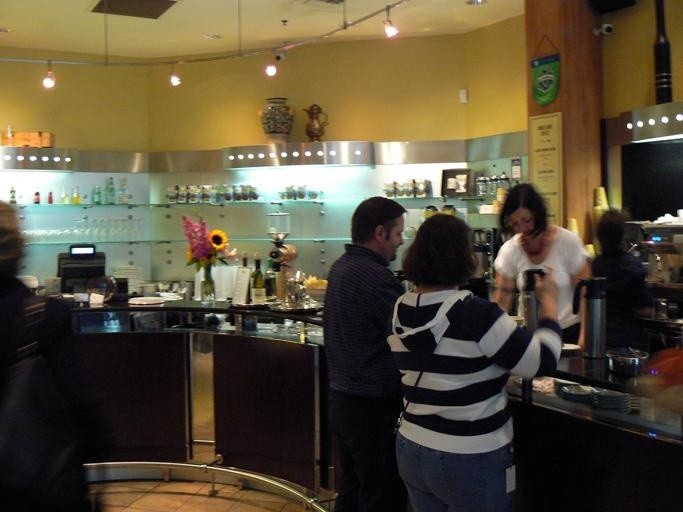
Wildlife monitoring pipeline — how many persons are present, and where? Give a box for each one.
[492,183,592,350]
[322,196,410,511]
[592,209,660,347]
[1,203,77,511]
[387,214,563,512]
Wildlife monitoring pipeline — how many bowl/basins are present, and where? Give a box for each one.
[601,348,650,376]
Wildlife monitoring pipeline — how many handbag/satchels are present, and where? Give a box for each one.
[0,341,108,511]
[368,432,398,473]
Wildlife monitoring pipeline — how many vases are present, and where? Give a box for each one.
[258,98,294,143]
[201,282,216,304]
[183,214,237,304]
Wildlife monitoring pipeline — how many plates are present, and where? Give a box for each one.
[553,379,640,414]
[117,267,157,297]
[128,297,165,305]
[562,343,580,352]
[162,291,183,301]
[269,302,325,314]
[231,302,269,309]
[305,288,328,301]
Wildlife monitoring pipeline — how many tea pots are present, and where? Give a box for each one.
[572,276,610,359]
[519,269,546,330]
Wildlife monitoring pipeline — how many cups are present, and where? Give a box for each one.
[653,298,672,320]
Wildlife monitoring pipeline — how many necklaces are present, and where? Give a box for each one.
[414,287,441,293]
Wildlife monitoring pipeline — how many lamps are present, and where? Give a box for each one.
[0,0,402,88]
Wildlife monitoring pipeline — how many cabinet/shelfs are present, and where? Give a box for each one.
[375,129,528,292]
[630,224,683,353]
[1,140,375,290]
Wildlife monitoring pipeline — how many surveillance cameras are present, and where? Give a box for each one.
[595,22,615,39]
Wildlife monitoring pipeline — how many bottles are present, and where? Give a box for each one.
[243,251,248,266]
[475,172,512,196]
[286,269,306,308]
[249,252,273,302]
[9,177,129,204]
